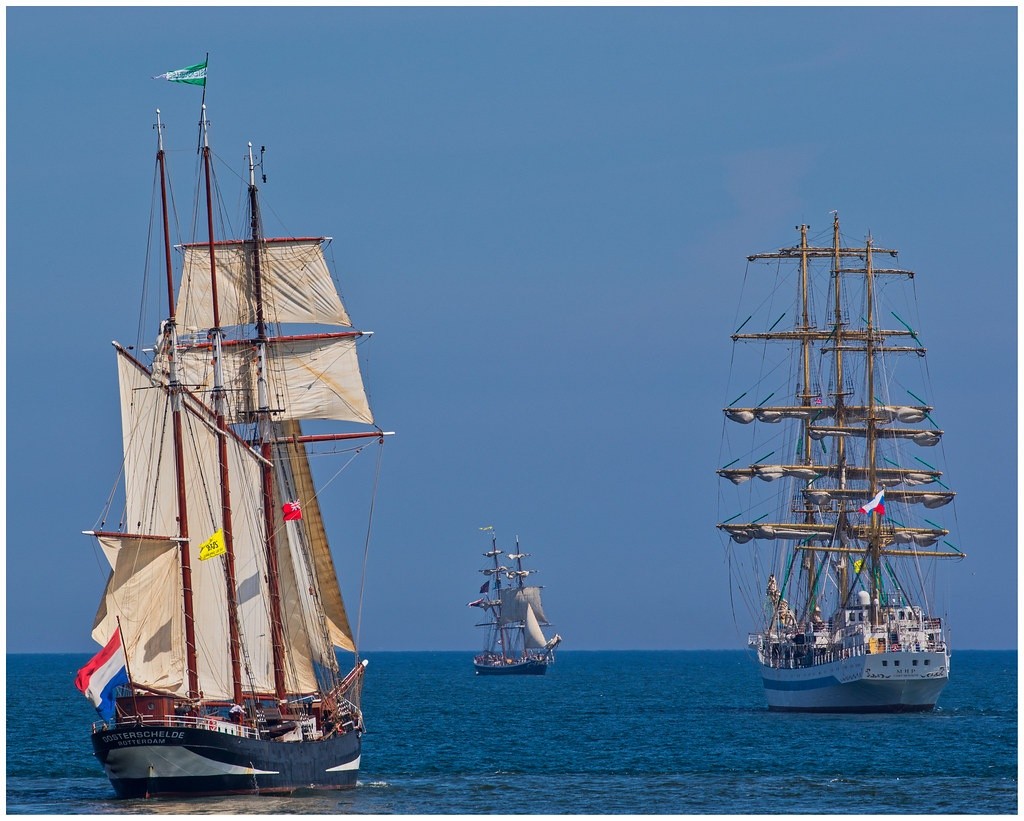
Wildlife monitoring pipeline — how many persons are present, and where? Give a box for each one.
[321,710,338,739]
[173,702,195,727]
[227,701,249,724]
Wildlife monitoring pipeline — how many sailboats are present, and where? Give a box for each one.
[718,206,969,714]
[465,526,563,676]
[79,102,396,802]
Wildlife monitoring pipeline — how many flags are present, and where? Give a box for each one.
[76,625,128,729]
[480,583,489,594]
[853,558,867,573]
[468,598,484,608]
[197,529,226,563]
[859,488,885,516]
[280,500,302,519]
